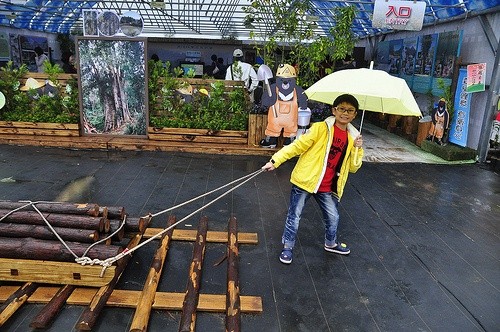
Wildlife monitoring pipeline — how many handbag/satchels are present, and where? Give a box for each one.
[213,66,219,74]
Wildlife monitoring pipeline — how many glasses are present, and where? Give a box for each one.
[333,106,356,114]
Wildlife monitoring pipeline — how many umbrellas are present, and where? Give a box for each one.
[302,60,424,167]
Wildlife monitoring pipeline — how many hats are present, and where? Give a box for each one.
[232,48,243,57]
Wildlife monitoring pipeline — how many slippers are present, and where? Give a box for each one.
[324,242,351,255]
[279,245,293,264]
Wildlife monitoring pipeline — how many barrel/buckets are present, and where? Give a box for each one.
[298,107,311,126]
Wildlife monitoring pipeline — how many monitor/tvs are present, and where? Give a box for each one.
[180,62,205,78]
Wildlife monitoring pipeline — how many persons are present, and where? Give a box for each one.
[224,48,259,105]
[34,46,52,74]
[61,51,77,74]
[205,54,226,79]
[261,94,364,264]
